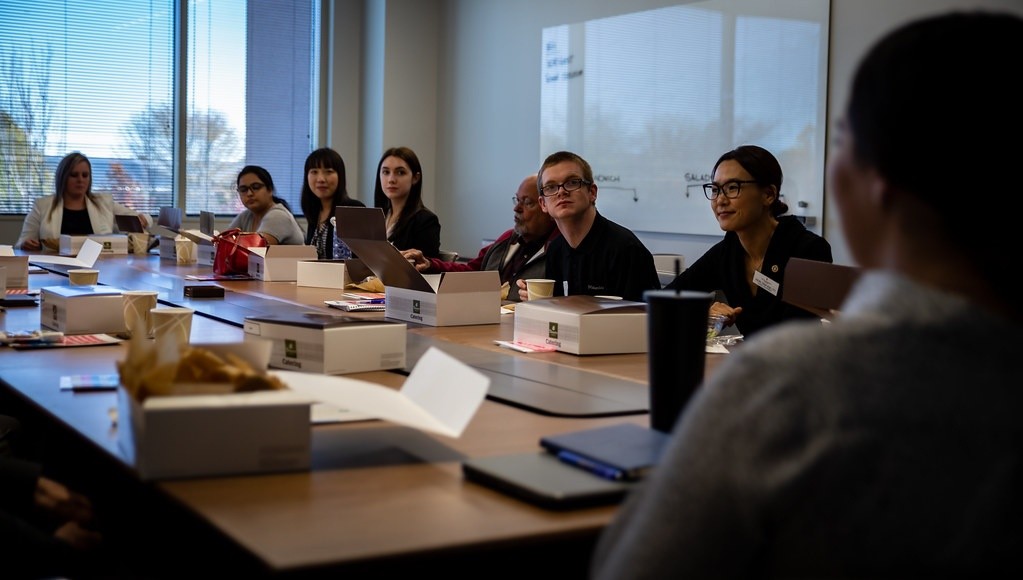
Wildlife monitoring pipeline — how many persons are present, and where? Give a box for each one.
[15,153,153,248]
[664,143,833,344]
[394,176,562,302]
[228,167,306,246]
[302,148,367,258]
[369,147,442,264]
[536,150,662,301]
[587,10,1023,580]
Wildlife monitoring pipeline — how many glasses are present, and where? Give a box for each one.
[541,178,590,196]
[237,182,266,194]
[702,179,753,200]
[511,197,537,209]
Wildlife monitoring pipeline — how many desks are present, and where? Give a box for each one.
[0,247,744,570]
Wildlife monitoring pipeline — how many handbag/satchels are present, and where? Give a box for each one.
[211,227,267,275]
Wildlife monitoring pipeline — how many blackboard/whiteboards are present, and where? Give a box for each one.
[538,0,831,239]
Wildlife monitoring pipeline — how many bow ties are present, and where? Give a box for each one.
[510,234,524,245]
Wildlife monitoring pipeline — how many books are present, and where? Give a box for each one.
[324,290,385,311]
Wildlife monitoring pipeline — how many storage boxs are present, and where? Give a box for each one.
[243,311,408,376]
[146,207,219,266]
[297,258,374,289]
[782,258,855,321]
[59,232,129,256]
[211,234,319,283]
[0,245,29,290]
[514,294,648,355]
[40,286,125,334]
[117,336,493,480]
[335,206,503,327]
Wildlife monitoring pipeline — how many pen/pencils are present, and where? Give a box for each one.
[556,450,623,482]
[356,298,385,305]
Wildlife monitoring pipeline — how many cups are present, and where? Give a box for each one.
[175,238,195,267]
[68,269,101,286]
[121,290,159,339]
[525,278,556,301]
[132,232,149,256]
[150,307,196,345]
[643,290,714,434]
